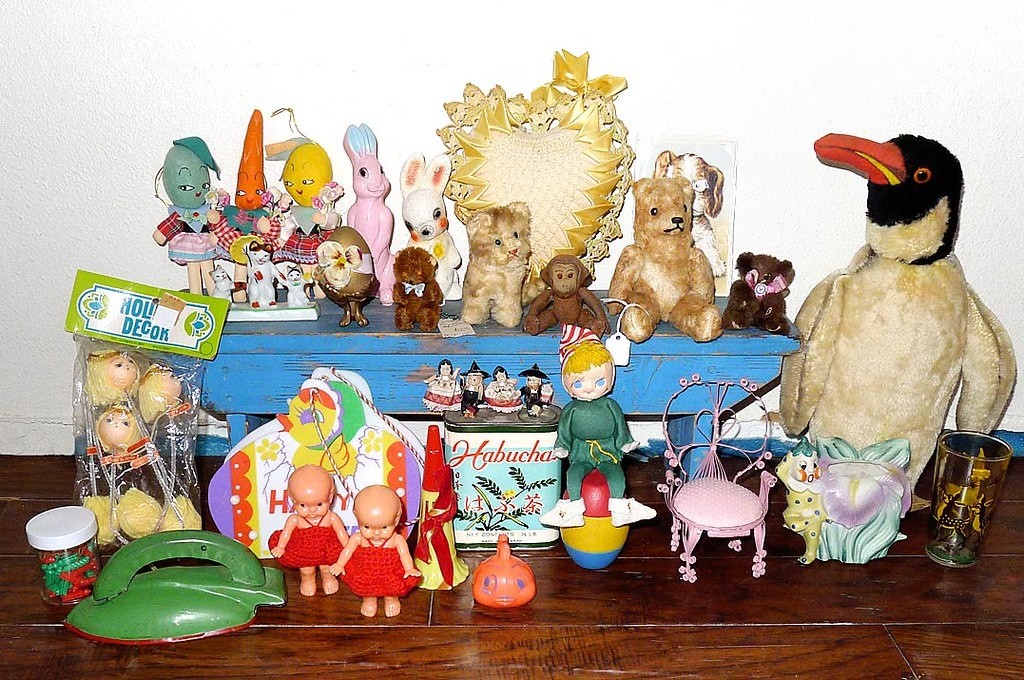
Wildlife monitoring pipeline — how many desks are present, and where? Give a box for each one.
[141,286,801,497]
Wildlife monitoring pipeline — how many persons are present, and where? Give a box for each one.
[264,465,348,598]
[539,322,659,530]
[328,484,423,618]
[420,358,552,420]
[67,345,205,542]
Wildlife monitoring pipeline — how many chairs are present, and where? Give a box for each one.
[656,375,780,585]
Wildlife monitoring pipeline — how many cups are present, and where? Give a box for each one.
[925,431,1012,568]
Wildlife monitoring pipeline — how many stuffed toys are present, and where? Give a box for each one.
[773,132,1018,493]
[156,109,797,338]
